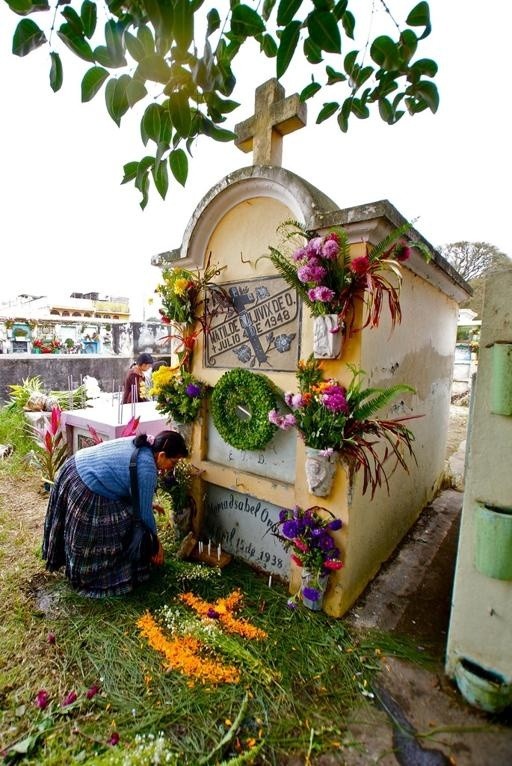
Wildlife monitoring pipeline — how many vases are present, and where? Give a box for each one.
[312,315,344,360]
[305,447,337,497]
[301,567,331,610]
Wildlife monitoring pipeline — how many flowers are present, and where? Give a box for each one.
[278,506,344,611]
[154,253,238,367]
[266,352,425,500]
[149,367,210,421]
[269,215,432,337]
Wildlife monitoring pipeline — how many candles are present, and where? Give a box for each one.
[196,537,222,562]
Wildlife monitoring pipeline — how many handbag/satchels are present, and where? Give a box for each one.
[123,518,159,569]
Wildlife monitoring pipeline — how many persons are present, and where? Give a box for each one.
[42,430,188,600]
[145,361,167,402]
[121,353,150,404]
